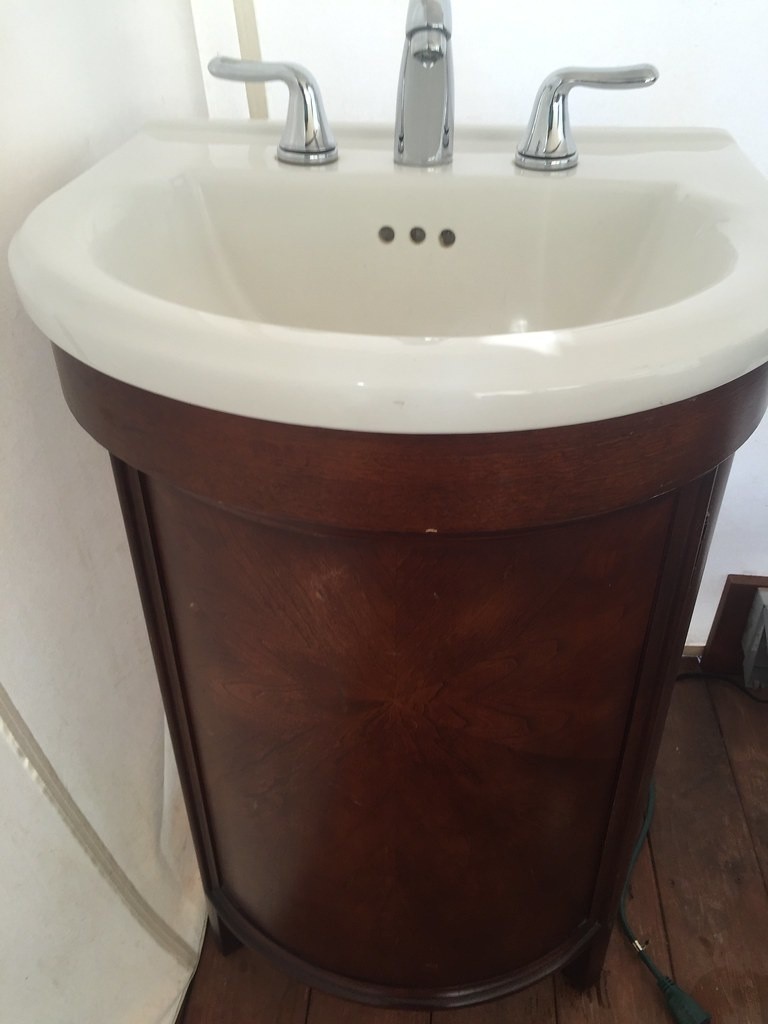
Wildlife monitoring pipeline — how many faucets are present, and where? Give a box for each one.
[390,0,455,170]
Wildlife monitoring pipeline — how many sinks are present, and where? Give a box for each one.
[4,115,764,437]
